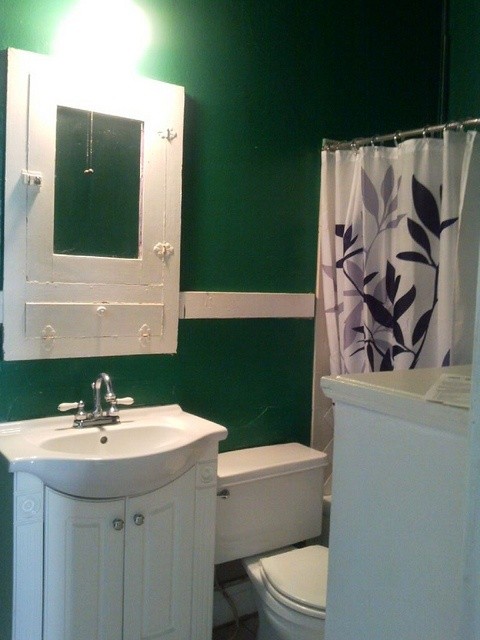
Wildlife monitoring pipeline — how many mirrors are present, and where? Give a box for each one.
[51,104,148,262]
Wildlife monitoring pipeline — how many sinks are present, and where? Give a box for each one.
[0,403,228,473]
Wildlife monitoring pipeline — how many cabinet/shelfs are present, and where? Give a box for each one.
[318,360,470,640]
[9,463,216,639]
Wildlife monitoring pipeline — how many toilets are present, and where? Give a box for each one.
[216,439,329,640]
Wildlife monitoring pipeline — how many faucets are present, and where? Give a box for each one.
[86,368,119,426]
